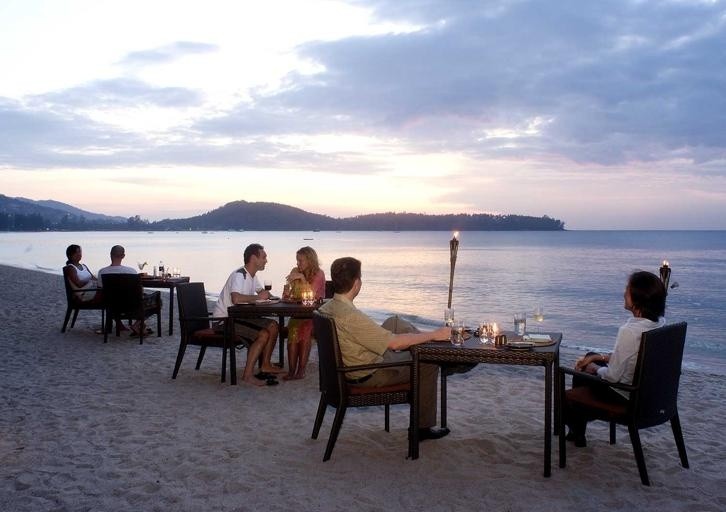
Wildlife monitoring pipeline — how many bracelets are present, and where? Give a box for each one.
[598,352,603,360]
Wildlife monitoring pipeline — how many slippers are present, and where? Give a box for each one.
[267,378,279,386]
[130,328,154,337]
[254,372,277,380]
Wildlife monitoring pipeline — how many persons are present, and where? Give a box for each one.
[64,244,130,331]
[282,246,325,380]
[209,245,287,386]
[562,271,669,447]
[319,258,479,442]
[96,246,153,337]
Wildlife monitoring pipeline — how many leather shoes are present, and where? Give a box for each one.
[408,427,449,441]
[447,363,478,375]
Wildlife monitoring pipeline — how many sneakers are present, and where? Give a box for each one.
[567,431,586,448]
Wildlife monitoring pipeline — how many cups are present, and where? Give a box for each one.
[444,307,454,328]
[264,280,272,293]
[305,290,313,303]
[533,303,544,336]
[479,320,488,345]
[451,320,465,347]
[488,321,498,343]
[301,289,308,304]
[513,311,527,337]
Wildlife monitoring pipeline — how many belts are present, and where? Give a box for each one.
[346,370,378,384]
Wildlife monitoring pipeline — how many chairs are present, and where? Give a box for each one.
[172,281,246,384]
[285,280,335,339]
[59,265,105,335]
[556,320,691,488]
[98,273,162,346]
[310,309,418,463]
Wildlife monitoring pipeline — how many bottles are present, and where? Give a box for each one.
[502,335,507,344]
[152,266,157,278]
[284,284,289,299]
[159,260,164,277]
[495,336,500,345]
